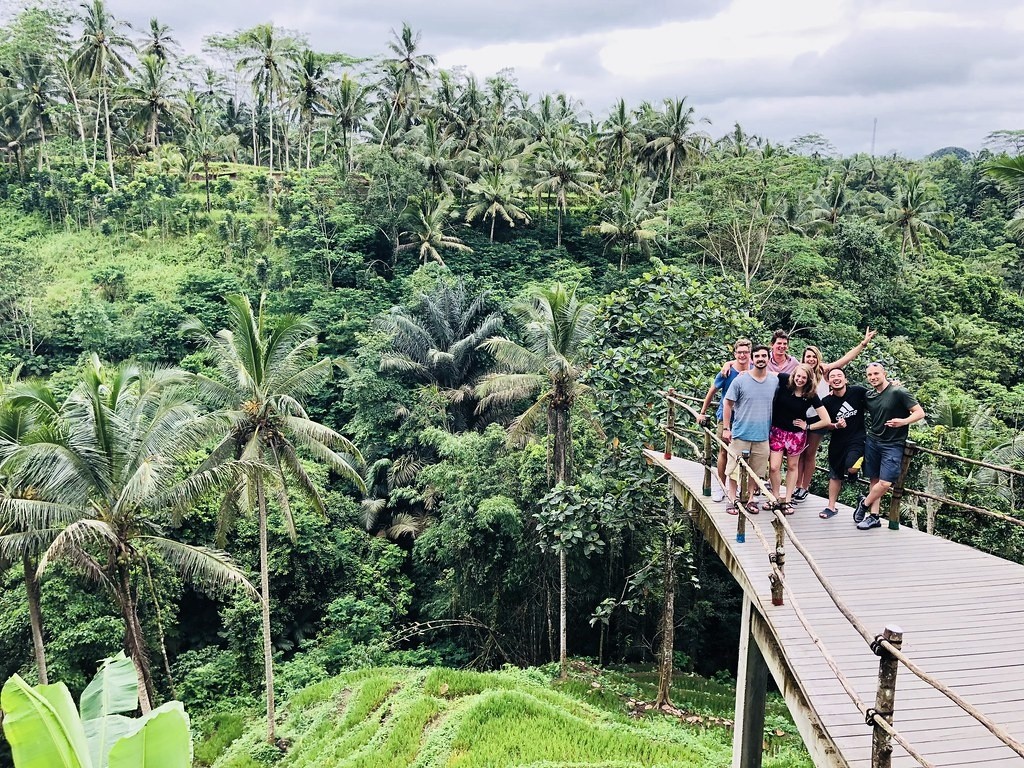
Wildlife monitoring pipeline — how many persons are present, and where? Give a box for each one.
[721,330,800,379]
[792,327,879,503]
[818,368,901,519]
[697,339,761,502]
[739,364,831,515]
[853,363,925,530]
[723,347,779,515]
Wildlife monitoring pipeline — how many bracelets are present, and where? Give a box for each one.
[861,343,867,347]
[806,424,810,430]
[723,428,730,430]
[700,413,705,415]
[834,424,838,429]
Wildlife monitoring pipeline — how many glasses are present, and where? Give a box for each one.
[735,351,749,355]
[865,363,878,371]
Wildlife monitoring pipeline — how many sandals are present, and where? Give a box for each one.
[753,485,772,495]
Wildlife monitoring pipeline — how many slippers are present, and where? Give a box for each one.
[726,500,759,515]
[849,472,859,484]
[763,500,794,515]
[819,507,839,520]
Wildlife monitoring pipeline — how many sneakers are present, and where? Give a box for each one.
[712,488,741,502]
[854,496,882,530]
[791,487,809,503]
